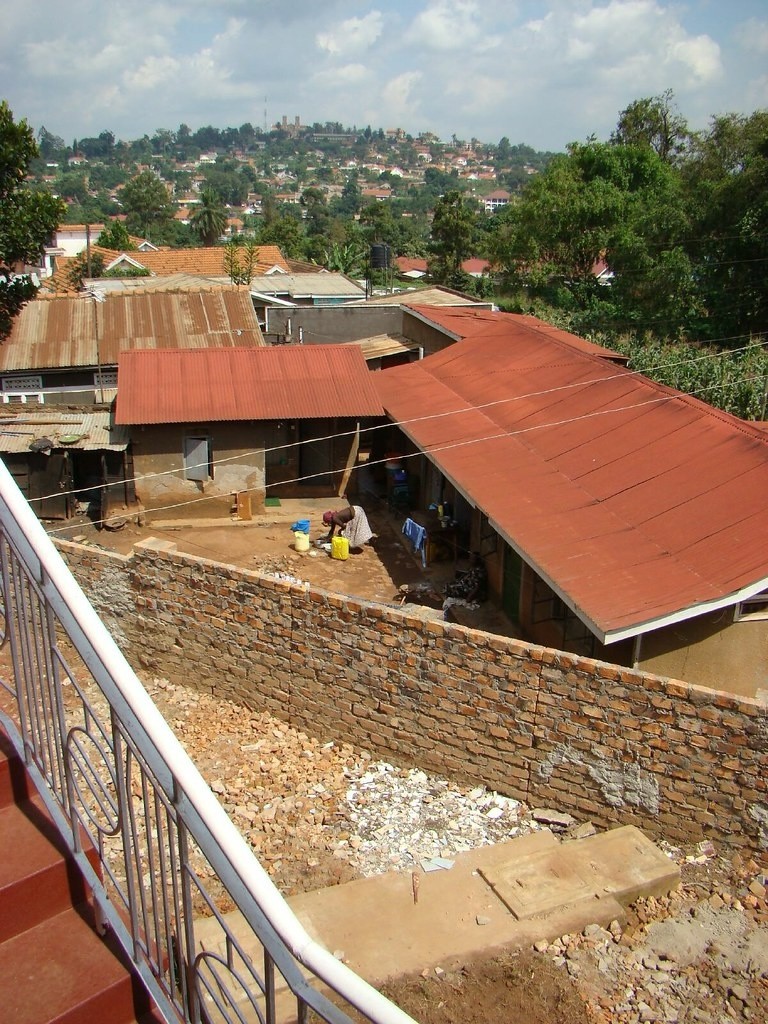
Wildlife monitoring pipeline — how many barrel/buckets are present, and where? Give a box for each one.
[296,519,310,533]
[331,536,349,560]
[294,531,309,551]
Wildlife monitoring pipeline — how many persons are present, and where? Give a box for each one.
[446,551,486,603]
[323,506,374,549]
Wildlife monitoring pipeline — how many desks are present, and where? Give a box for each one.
[410,508,460,567]
[384,466,420,520]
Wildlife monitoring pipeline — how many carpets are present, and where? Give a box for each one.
[265,497,283,507]
